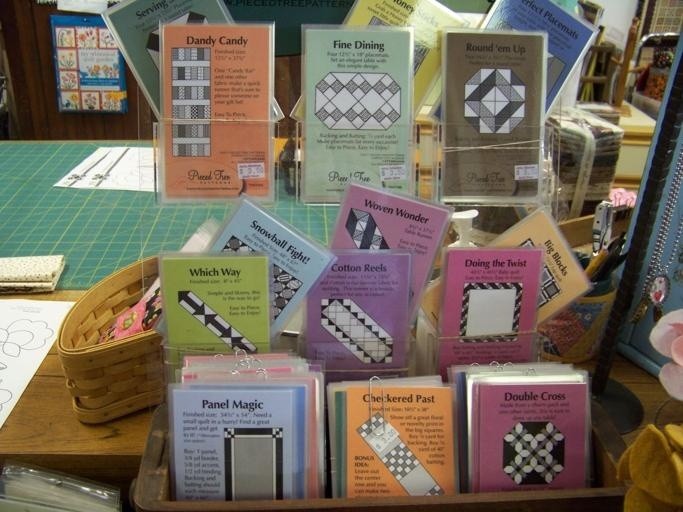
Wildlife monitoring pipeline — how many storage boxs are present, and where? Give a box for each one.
[303,341,417,383]
[133,397,627,511]
[153,125,275,205]
[614,101,655,188]
[417,331,540,375]
[161,343,278,385]
[435,126,552,205]
[294,123,419,210]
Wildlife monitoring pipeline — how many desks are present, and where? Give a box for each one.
[0,139,683,511]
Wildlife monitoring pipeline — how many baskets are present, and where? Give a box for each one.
[57,256,169,425]
